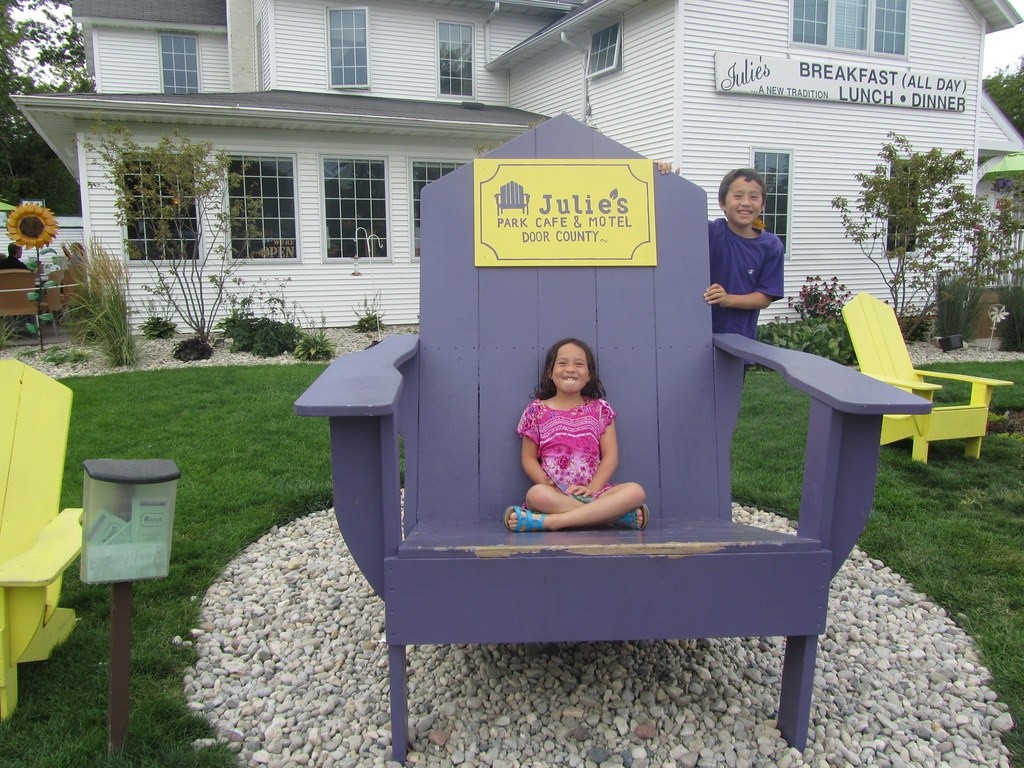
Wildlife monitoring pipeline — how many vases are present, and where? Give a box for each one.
[938,333,962,353]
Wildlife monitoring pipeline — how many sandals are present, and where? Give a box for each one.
[503,506,546,532]
[614,503,649,530]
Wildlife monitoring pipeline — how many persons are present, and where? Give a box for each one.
[62,242,85,264]
[656,161,785,382]
[0,243,32,270]
[503,338,650,532]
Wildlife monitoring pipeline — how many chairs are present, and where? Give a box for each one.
[38,269,66,337]
[0,355,92,721]
[0,269,43,341]
[844,290,1014,469]
[61,267,81,323]
[291,98,938,761]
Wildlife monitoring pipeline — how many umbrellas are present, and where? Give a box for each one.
[977,154,1024,219]
